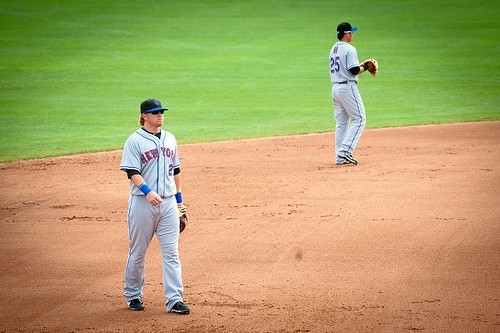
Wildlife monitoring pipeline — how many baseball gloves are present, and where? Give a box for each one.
[178,207,189,233]
[360,58,379,76]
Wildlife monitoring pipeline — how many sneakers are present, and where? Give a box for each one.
[166,300,190,315]
[338,154,358,165]
[127,298,144,311]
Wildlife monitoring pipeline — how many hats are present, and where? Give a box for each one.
[141,99,168,114]
[337,22,357,34]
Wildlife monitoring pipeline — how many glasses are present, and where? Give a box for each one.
[147,109,164,114]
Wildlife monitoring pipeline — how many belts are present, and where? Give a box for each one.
[339,81,347,84]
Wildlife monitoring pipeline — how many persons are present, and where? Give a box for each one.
[329,22,378,165]
[119,98,191,313]
